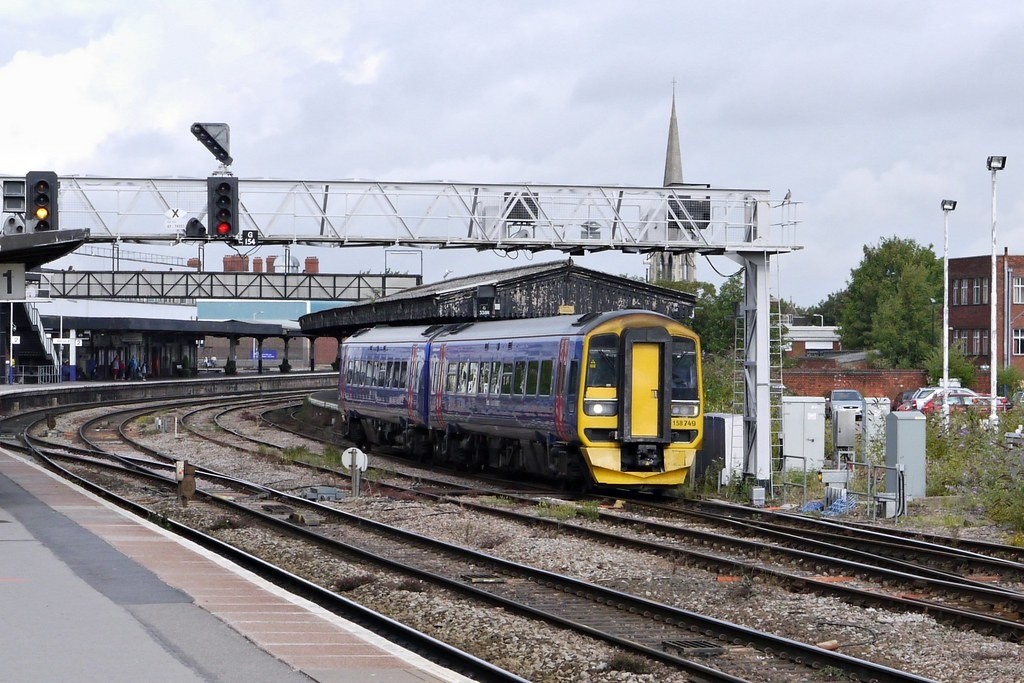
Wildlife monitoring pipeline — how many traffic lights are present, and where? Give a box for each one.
[24,170,60,233]
[2,179,26,213]
[2,216,26,236]
[185,216,206,238]
[204,175,239,238]
[189,120,234,166]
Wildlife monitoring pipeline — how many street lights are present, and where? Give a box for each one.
[929,296,937,358]
[940,198,957,447]
[812,313,823,326]
[984,155,1008,437]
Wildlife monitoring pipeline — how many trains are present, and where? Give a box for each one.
[336,308,706,497]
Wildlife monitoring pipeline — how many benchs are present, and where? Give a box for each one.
[197,368,225,373]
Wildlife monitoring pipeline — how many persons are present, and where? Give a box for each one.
[113,355,138,382]
[142,362,147,381]
[204,355,216,367]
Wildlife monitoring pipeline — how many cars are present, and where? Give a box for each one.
[891,384,1024,418]
[824,389,863,421]
